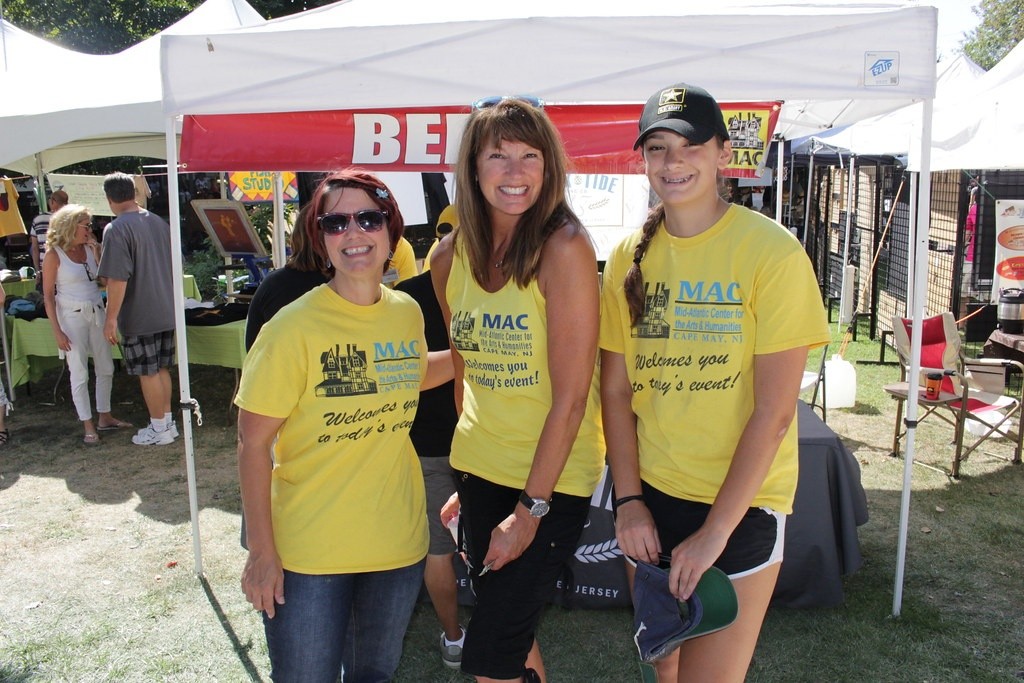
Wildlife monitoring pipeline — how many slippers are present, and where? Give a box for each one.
[83,434,100,443]
[96,418,134,430]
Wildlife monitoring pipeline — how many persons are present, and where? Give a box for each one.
[148,176,160,210]
[30,190,68,291]
[208,179,219,199]
[245,204,546,683]
[430,95,606,683]
[958,187,978,330]
[179,175,191,220]
[97,172,185,445]
[42,204,133,442]
[598,84,833,683]
[194,173,208,199]
[0,282,14,446]
[234,170,455,683]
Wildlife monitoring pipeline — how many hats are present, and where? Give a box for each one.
[435,203,463,238]
[630,550,740,662]
[633,81,730,152]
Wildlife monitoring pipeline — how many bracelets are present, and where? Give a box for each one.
[35,270,41,275]
[616,495,644,506]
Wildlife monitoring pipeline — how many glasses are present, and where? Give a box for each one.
[75,221,93,230]
[48,196,57,202]
[317,209,389,235]
[83,262,94,281]
[471,95,545,113]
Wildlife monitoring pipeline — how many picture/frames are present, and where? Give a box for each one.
[190,199,266,257]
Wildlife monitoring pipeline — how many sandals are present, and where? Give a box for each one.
[0,429,9,446]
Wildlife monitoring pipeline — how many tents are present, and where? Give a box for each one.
[0,0,285,401]
[790,35,1024,419]
[160,0,939,615]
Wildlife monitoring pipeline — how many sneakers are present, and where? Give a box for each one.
[137,420,180,439]
[440,621,467,666]
[132,427,175,446]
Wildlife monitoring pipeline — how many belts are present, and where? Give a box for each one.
[76,304,101,313]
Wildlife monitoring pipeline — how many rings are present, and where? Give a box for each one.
[109,336,112,339]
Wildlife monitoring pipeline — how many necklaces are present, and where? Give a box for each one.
[494,261,502,268]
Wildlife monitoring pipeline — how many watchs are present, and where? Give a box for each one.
[519,489,553,518]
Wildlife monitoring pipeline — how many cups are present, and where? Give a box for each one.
[925,372,945,403]
[447,509,472,567]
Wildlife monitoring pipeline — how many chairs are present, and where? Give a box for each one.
[886,312,1024,479]
[800,344,829,422]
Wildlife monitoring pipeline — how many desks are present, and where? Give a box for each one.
[1,275,250,430]
[449,397,870,614]
[982,330,1024,395]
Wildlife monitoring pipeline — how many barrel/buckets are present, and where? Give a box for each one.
[819,352,858,409]
[998,288,1024,335]
